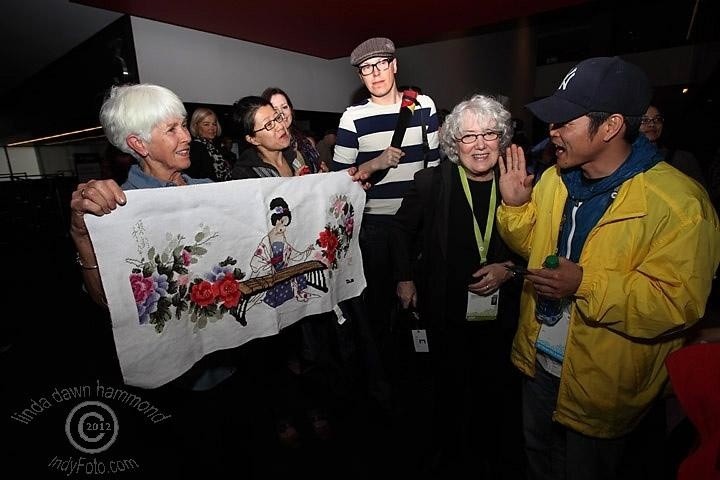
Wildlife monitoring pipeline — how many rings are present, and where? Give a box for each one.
[486,285,490,290]
[80,188,86,199]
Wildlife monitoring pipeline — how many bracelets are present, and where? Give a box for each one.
[73,250,98,270]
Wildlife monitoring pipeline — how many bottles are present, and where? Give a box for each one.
[535,255,563,326]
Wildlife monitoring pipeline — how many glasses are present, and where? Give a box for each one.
[643,116,665,124]
[255,112,285,132]
[456,132,502,144]
[357,57,393,76]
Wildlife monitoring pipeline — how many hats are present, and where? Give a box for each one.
[350,37,396,66]
[525,57,651,124]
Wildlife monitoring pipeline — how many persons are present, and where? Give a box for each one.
[70,82,240,480]
[259,86,333,378]
[495,56,720,480]
[386,92,532,480]
[316,128,337,170]
[637,98,712,200]
[329,37,448,417]
[183,107,236,182]
[230,95,373,449]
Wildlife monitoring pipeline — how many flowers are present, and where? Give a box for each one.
[129,224,247,333]
[315,193,355,277]
